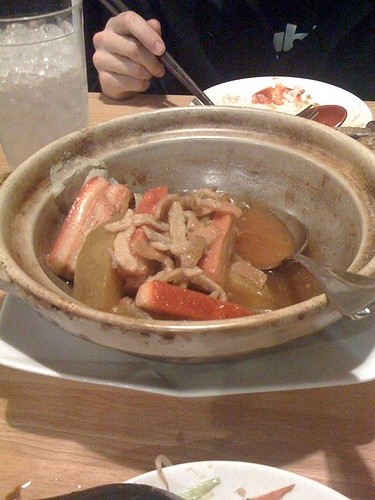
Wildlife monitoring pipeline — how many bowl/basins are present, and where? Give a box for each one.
[2,105,374,362]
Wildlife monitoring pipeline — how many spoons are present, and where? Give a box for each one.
[304,103,348,129]
[242,202,374,323]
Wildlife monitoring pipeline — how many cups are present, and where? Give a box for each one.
[2,1,92,173]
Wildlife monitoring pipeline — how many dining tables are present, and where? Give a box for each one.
[0,94,375,500]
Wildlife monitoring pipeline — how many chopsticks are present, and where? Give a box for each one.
[103,0,216,108]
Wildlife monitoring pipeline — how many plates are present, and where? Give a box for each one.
[189,76,373,137]
[0,293,374,396]
[47,461,351,500]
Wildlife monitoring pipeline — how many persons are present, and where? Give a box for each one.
[83,0,375,102]
